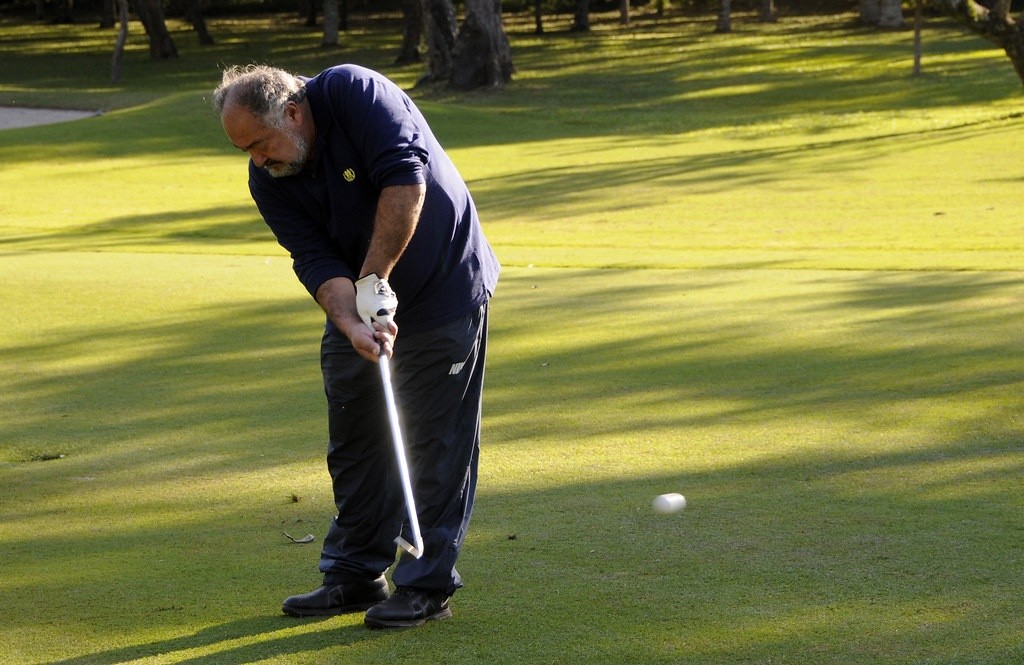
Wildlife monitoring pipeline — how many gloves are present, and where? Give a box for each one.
[355,273,398,345]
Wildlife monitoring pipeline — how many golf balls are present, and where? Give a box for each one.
[652,492,686,514]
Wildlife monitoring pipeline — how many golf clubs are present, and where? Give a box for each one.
[375,331,425,559]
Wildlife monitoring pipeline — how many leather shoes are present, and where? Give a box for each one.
[364,588,452,627]
[281,574,389,616]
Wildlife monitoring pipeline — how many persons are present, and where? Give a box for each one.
[212,63,501,628]
